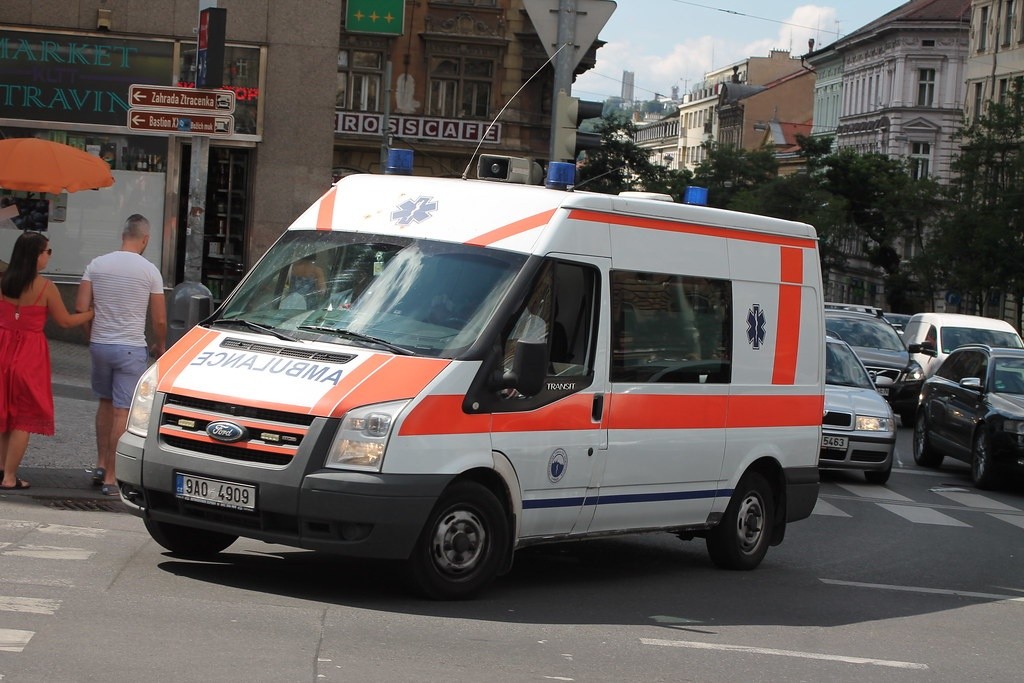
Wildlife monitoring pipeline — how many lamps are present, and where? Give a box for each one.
[754,121,766,132]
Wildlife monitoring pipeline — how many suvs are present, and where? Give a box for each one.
[822,302,927,428]
[815,328,896,487]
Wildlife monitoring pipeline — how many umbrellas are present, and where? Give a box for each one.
[0,138,114,232]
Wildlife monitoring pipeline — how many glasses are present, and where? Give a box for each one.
[41,247,54,256]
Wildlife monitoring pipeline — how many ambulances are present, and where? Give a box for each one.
[112,146,826,601]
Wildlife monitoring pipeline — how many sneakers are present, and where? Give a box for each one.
[90,467,105,485]
[100,480,122,496]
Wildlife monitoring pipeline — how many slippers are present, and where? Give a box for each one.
[0,471,32,490]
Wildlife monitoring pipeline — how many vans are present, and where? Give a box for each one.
[902,312,1024,379]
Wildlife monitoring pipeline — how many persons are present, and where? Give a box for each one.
[0,232,94,488]
[505,309,546,372]
[273,242,325,320]
[76,215,166,496]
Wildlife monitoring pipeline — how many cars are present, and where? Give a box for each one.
[912,342,1024,489]
[881,312,911,334]
